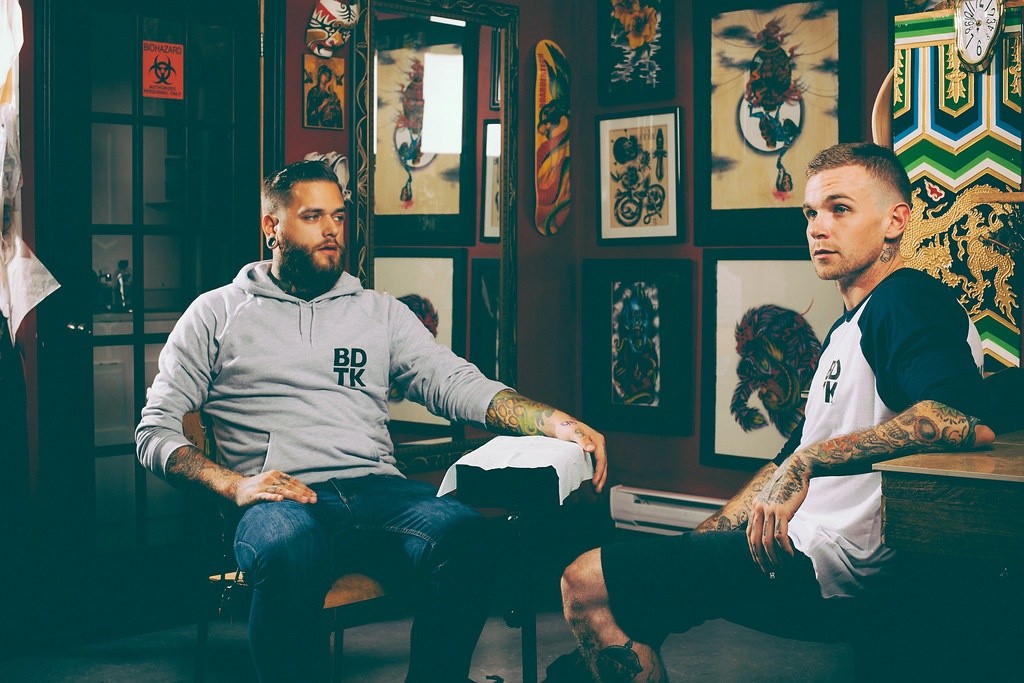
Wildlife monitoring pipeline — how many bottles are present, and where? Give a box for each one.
[112,261,132,313]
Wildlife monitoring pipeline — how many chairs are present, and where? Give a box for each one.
[183,408,538,683]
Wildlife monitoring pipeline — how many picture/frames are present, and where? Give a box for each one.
[593,106,687,247]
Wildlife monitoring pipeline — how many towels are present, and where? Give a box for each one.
[436,433,595,507]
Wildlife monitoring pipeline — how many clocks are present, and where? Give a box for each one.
[953,0,1005,75]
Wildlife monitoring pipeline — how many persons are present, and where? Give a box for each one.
[134,160,607,683]
[560,140,1002,683]
